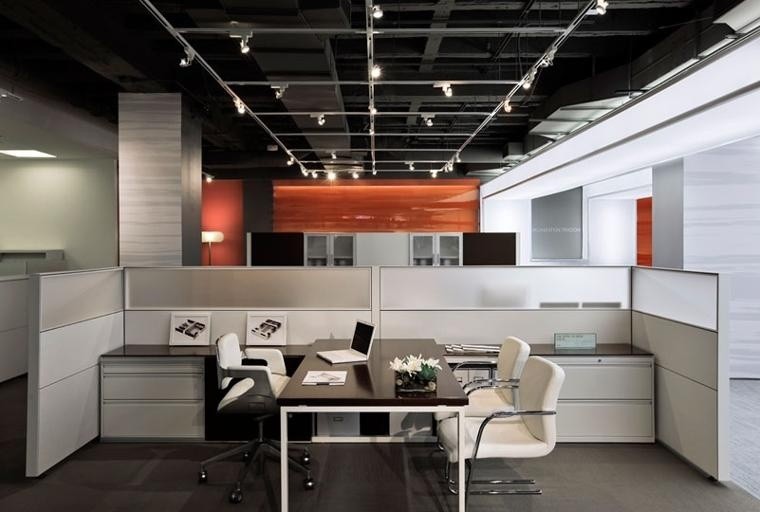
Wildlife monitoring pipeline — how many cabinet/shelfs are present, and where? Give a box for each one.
[437,365,493,421]
[208,356,311,442]
[410,232,463,266]
[0,250,65,383]
[98,357,206,443]
[316,412,434,444]
[304,231,355,267]
[554,356,655,442]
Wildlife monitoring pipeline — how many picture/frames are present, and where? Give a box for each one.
[168,313,211,346]
[246,312,286,345]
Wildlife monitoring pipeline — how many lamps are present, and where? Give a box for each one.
[374,5,383,19]
[596,1,608,15]
[179,57,190,66]
[241,39,250,54]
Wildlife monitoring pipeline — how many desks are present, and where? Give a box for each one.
[277,337,469,512]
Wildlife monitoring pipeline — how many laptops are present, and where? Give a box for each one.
[317,320,376,365]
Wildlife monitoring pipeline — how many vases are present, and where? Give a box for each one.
[395,373,436,397]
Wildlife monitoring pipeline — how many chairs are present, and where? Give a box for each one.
[434,337,531,475]
[200,332,315,504]
[438,356,565,512]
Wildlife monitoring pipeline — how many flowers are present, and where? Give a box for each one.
[389,355,443,379]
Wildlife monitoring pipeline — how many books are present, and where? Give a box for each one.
[302,370,348,385]
